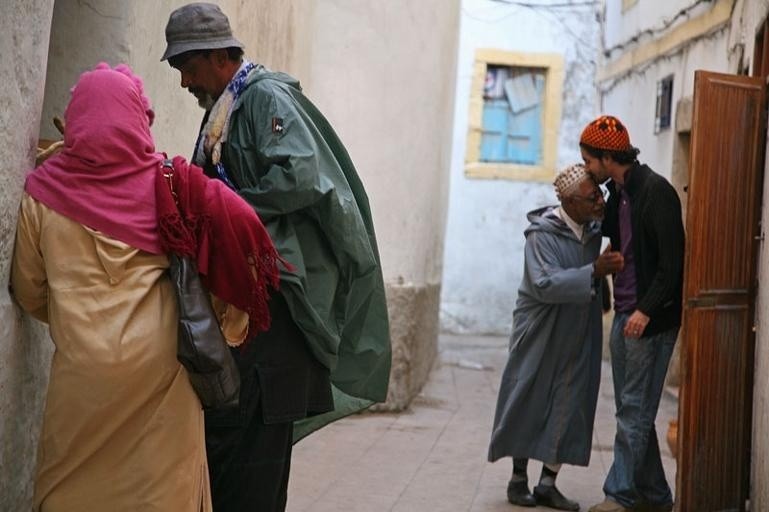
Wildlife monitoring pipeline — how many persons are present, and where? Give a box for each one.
[9,60,294,512]
[488,163,626,512]
[161,3,392,512]
[579,115,688,512]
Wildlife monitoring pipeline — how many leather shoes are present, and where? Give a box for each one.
[507,480,537,507]
[533,483,579,511]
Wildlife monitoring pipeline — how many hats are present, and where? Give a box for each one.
[160,3,244,62]
[579,115,631,153]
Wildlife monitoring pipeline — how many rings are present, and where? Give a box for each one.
[633,330,639,335]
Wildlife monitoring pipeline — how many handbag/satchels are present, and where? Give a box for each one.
[169,254,241,413]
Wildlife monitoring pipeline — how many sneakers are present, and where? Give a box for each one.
[588,496,632,511]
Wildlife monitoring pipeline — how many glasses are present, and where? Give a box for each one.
[573,189,607,204]
